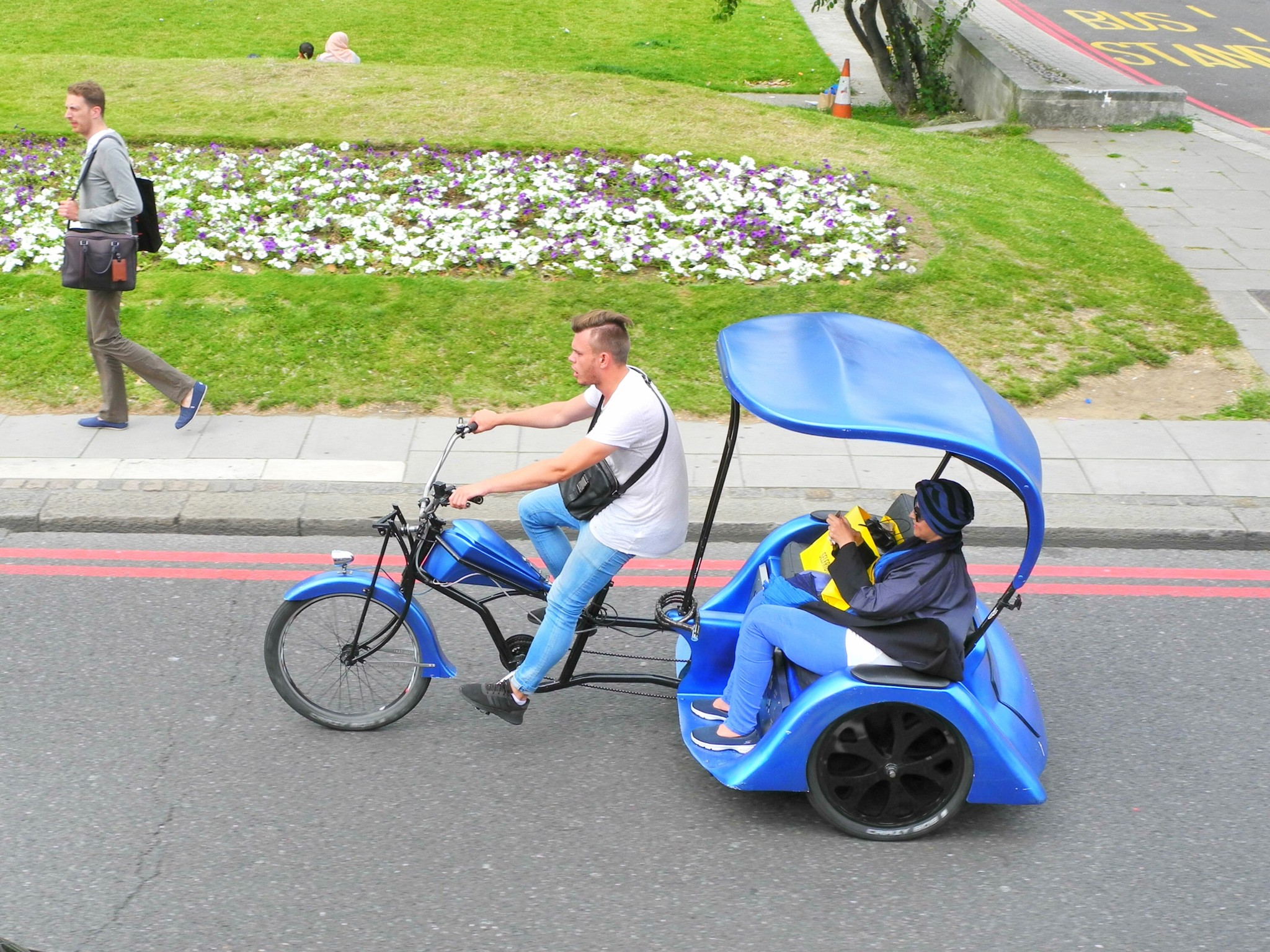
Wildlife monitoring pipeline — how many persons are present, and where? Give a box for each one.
[57,80,208,430]
[690,478,975,754]
[449,309,689,725]
[296,42,314,60]
[315,32,360,63]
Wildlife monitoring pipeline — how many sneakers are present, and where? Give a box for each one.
[528,607,598,636]
[691,697,728,721]
[691,724,759,754]
[460,680,529,725]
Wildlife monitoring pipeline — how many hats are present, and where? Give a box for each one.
[915,479,974,537]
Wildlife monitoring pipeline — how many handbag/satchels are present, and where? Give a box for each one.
[115,150,163,254]
[800,506,905,611]
[559,459,621,522]
[61,230,137,291]
[859,516,911,555]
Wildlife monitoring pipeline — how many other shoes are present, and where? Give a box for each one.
[77,416,128,430]
[175,381,207,430]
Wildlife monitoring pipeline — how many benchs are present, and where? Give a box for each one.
[780,493,976,690]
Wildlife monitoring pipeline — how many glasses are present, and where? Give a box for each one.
[914,496,924,522]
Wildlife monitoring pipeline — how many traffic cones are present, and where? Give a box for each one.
[829,59,852,118]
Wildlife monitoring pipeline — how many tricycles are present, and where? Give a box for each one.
[261,310,1051,844]
